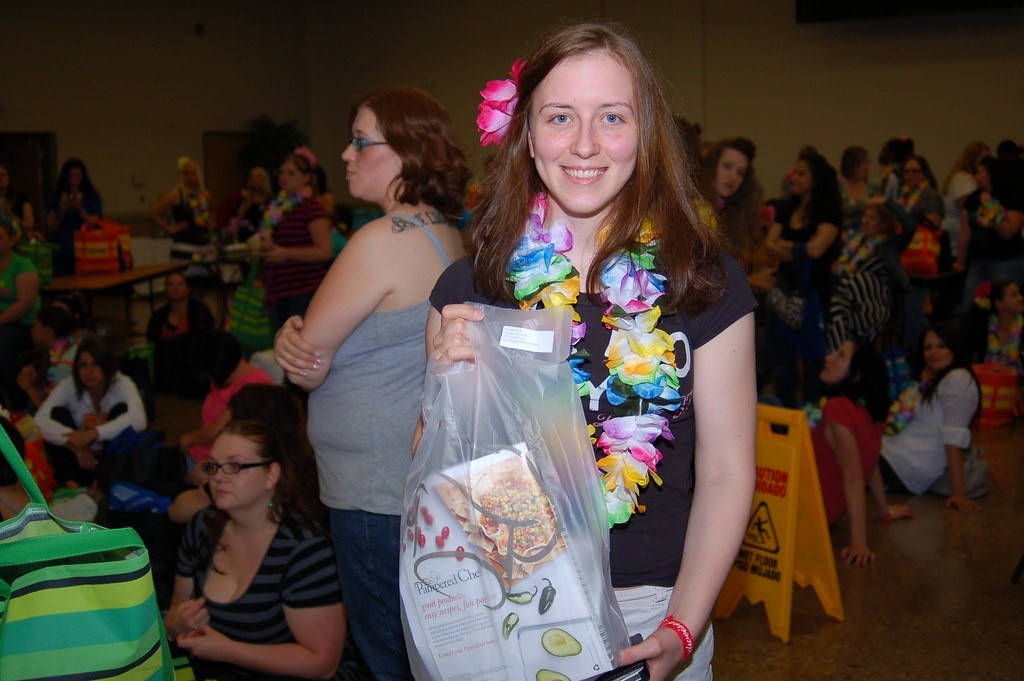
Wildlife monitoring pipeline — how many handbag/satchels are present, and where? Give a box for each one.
[73,216,132,273]
[975,361,1021,428]
[229,256,276,350]
[899,225,940,275]
[401,299,643,681]
[126,341,154,391]
[15,236,61,288]
[1,423,176,681]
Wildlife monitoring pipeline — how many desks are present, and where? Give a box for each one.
[36,261,190,359]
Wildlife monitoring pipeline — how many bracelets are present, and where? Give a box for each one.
[657,614,695,668]
[877,506,893,518]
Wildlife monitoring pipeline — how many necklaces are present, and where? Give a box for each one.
[839,232,888,273]
[503,192,683,526]
[800,396,829,430]
[882,368,936,436]
[257,183,312,250]
[40,327,89,396]
[177,183,209,227]
[987,310,1024,368]
[893,180,928,233]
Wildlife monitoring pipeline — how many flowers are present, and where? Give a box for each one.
[476,60,525,144]
[294,147,318,168]
[176,156,189,171]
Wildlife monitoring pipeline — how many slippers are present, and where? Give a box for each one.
[346,134,389,152]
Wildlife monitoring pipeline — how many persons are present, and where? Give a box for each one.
[0,23,1024,681]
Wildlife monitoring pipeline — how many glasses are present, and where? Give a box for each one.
[200,459,271,475]
[900,167,923,176]
